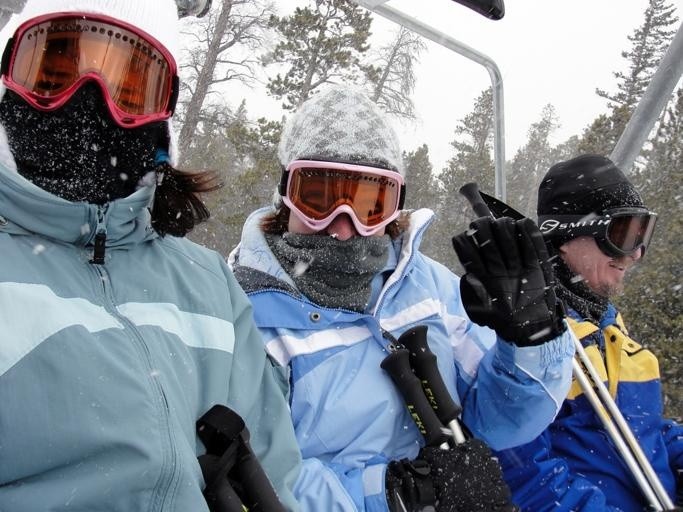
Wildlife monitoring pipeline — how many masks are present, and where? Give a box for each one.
[1,82,159,202]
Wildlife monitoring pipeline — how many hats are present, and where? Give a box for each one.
[538,153,644,256]
[1,0,178,67]
[277,86,407,182]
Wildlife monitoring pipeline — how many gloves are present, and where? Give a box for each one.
[451,214,568,347]
[386,440,515,512]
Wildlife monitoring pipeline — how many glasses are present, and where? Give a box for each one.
[541,210,660,259]
[278,157,405,239]
[0,11,178,127]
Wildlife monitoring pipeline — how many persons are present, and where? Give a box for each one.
[229,84,577,512]
[0,0,303,512]
[497,154,683,512]
